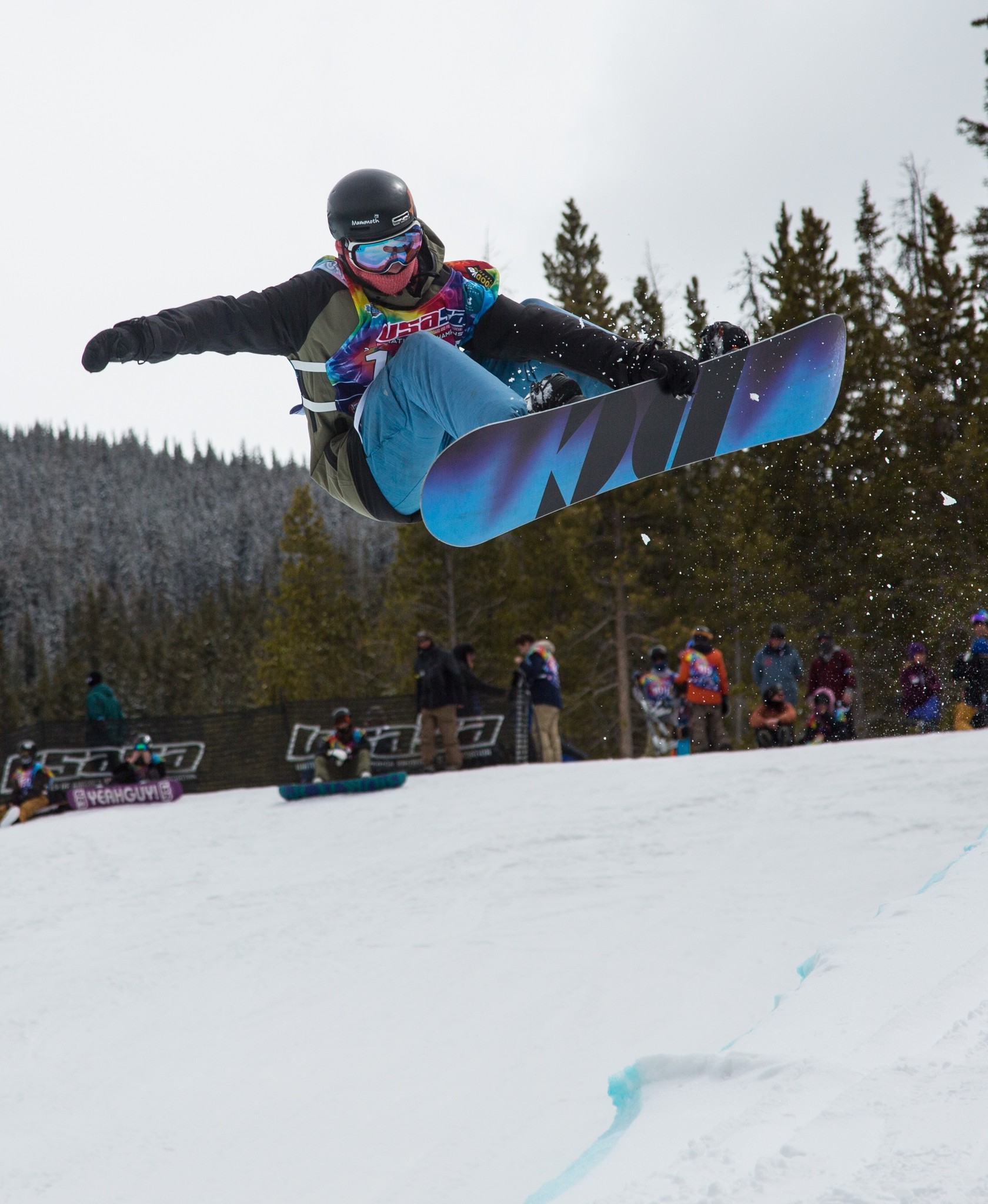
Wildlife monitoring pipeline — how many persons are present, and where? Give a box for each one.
[411,633,466,773]
[109,734,167,786]
[900,645,940,731]
[313,706,372,783]
[507,632,564,761]
[751,624,859,748]
[451,644,510,770]
[951,612,988,729]
[81,670,124,749]
[79,169,754,524]
[0,739,56,828]
[635,618,734,759]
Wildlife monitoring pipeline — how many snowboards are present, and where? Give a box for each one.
[277,771,406,802]
[416,307,853,552]
[67,779,184,810]
[513,679,529,762]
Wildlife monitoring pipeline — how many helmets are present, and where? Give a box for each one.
[332,709,350,724]
[692,627,713,642]
[19,740,35,754]
[650,645,669,662]
[814,630,835,652]
[908,643,926,662]
[328,169,414,242]
[136,734,153,751]
[84,668,103,687]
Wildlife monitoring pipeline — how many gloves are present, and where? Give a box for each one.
[721,699,731,718]
[636,345,699,397]
[81,318,142,373]
[330,749,349,769]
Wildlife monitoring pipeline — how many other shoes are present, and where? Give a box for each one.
[528,372,580,413]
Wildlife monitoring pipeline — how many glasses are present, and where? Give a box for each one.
[343,232,425,274]
[136,745,148,752]
[970,614,986,624]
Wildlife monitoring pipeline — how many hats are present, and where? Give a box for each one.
[814,694,829,705]
[763,685,781,704]
[768,624,786,639]
